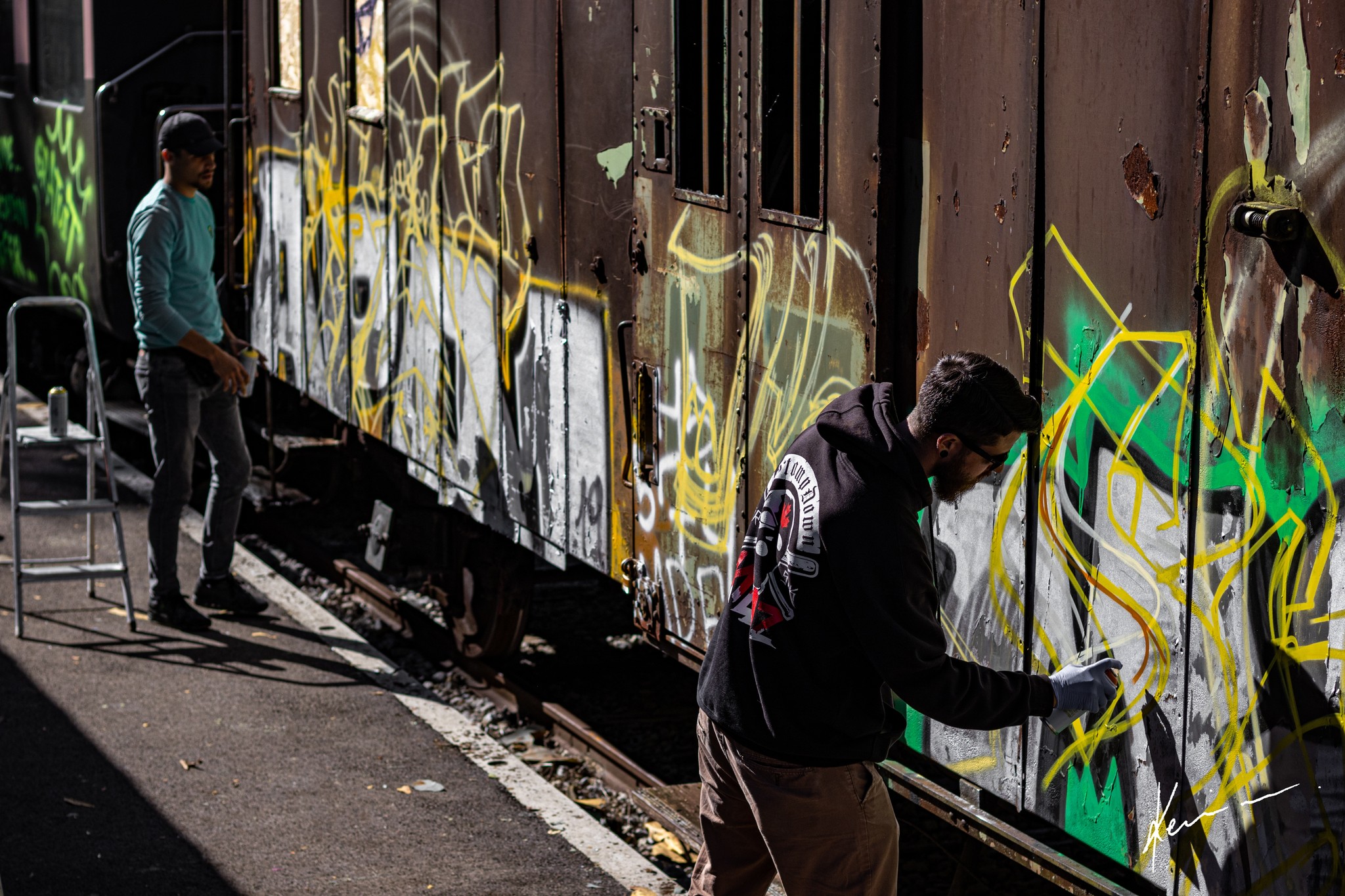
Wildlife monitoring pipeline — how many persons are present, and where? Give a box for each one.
[684,349,1124,896]
[127,111,272,638]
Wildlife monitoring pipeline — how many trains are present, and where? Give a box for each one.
[0,2,1345,895]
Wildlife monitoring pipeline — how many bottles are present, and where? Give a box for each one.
[235,345,259,397]
[1038,666,1121,735]
[48,386,68,437]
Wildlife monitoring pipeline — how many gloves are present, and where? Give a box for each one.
[1047,658,1123,714]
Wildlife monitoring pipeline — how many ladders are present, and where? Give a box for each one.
[0,295,137,638]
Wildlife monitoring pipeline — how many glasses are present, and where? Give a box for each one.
[949,430,1011,472]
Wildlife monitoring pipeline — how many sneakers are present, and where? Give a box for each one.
[191,573,270,613]
[148,595,212,631]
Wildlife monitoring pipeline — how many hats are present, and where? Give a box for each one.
[158,111,230,158]
